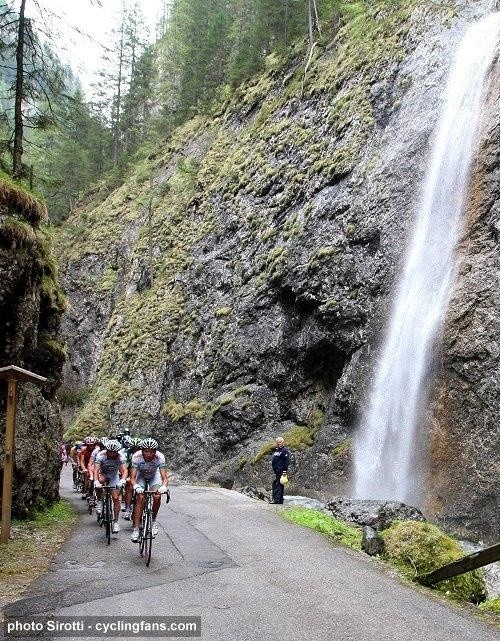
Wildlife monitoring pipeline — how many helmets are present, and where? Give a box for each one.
[75,435,158,453]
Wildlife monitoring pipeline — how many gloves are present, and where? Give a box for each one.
[90,476,167,494]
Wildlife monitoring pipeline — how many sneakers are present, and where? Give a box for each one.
[88,493,132,520]
[152,523,158,534]
[112,523,120,533]
[131,529,140,541]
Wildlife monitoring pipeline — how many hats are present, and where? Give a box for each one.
[280,474,288,485]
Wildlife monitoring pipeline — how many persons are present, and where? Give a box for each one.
[269,437,289,504]
[59,432,170,542]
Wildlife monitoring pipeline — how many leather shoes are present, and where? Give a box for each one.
[270,501,283,504]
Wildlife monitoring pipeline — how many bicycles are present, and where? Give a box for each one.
[72,461,123,546]
[137,490,171,567]
